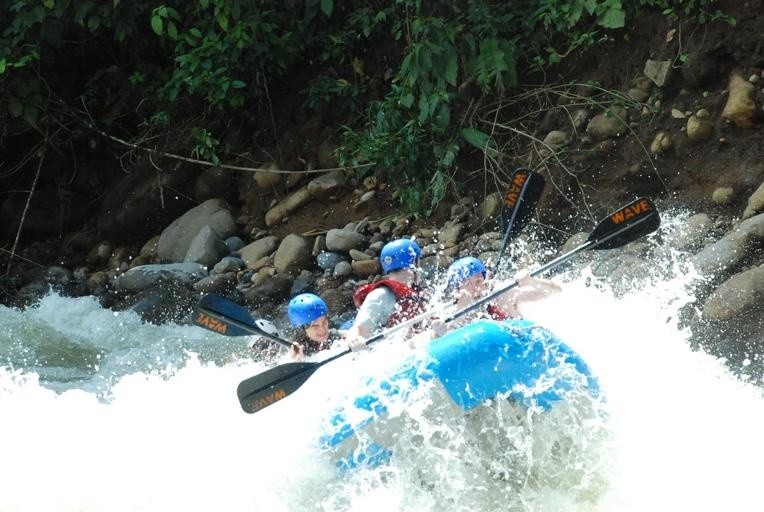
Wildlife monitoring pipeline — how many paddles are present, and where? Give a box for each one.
[489,167,546,277]
[444,197,660,322]
[191,292,315,357]
[237,300,458,413]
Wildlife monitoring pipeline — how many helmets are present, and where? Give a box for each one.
[447,256,486,289]
[287,292,327,326]
[381,239,420,273]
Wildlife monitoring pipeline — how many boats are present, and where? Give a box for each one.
[316,318,611,507]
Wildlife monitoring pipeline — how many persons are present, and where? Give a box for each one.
[245,317,283,369]
[432,256,513,339]
[286,292,350,362]
[352,238,431,339]
